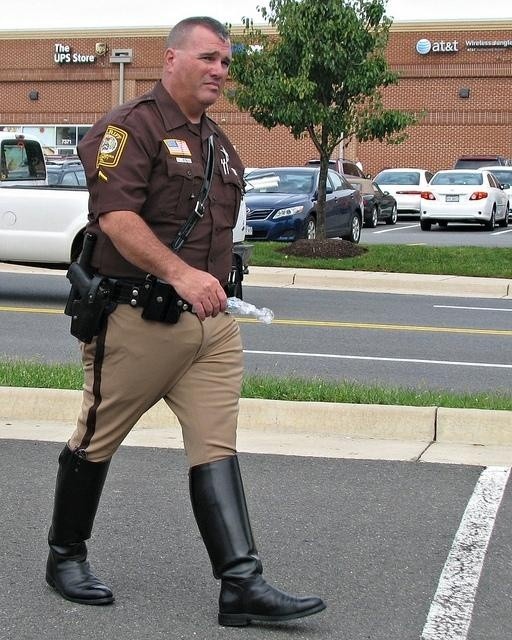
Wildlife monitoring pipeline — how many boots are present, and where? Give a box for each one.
[45,443,116,606]
[188,454,326,628]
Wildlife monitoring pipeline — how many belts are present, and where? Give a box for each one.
[103,274,189,312]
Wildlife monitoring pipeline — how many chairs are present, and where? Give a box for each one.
[267,179,311,193]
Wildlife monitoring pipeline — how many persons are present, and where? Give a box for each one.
[46,17,329,627]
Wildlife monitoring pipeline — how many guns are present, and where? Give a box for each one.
[66,261,111,344]
